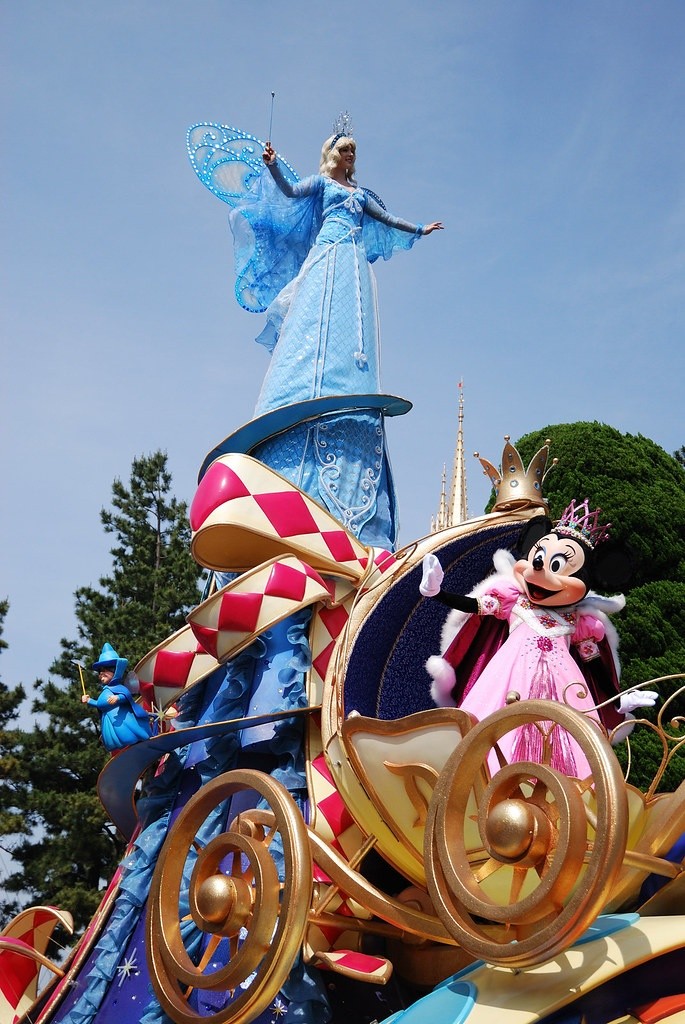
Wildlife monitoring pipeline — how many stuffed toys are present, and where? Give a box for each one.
[422,497,658,788]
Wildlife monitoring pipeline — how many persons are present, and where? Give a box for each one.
[81,643,156,750]
[228,113,446,555]
